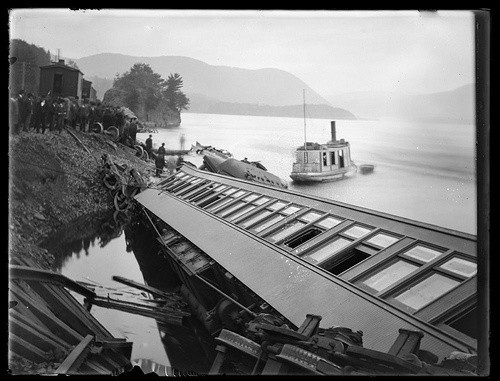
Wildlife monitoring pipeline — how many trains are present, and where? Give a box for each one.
[133,154,479,380]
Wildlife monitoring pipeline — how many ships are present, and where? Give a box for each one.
[290,88,357,182]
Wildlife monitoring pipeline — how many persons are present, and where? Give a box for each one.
[159,143,165,161]
[176,153,183,166]
[155,153,165,178]
[145,134,152,158]
[8,89,140,145]
